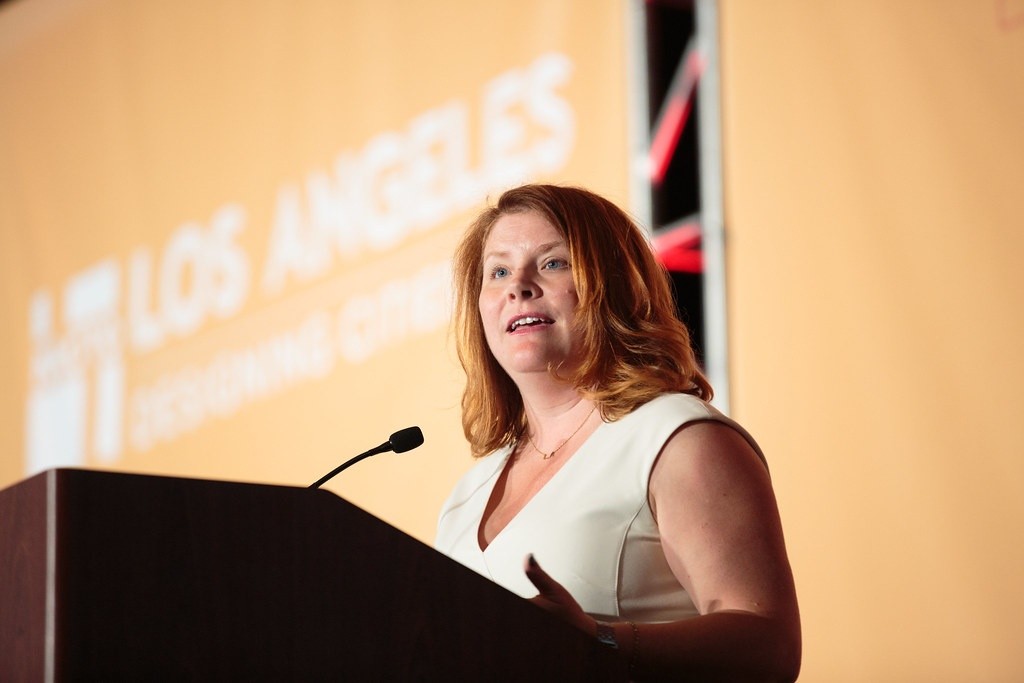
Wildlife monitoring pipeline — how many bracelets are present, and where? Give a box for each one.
[583,621,624,669]
[626,622,640,672]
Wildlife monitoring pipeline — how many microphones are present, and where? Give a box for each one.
[308,426,424,489]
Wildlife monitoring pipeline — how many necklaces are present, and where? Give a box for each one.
[526,406,595,460]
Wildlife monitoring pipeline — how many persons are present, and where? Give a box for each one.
[436,184,801,683]
